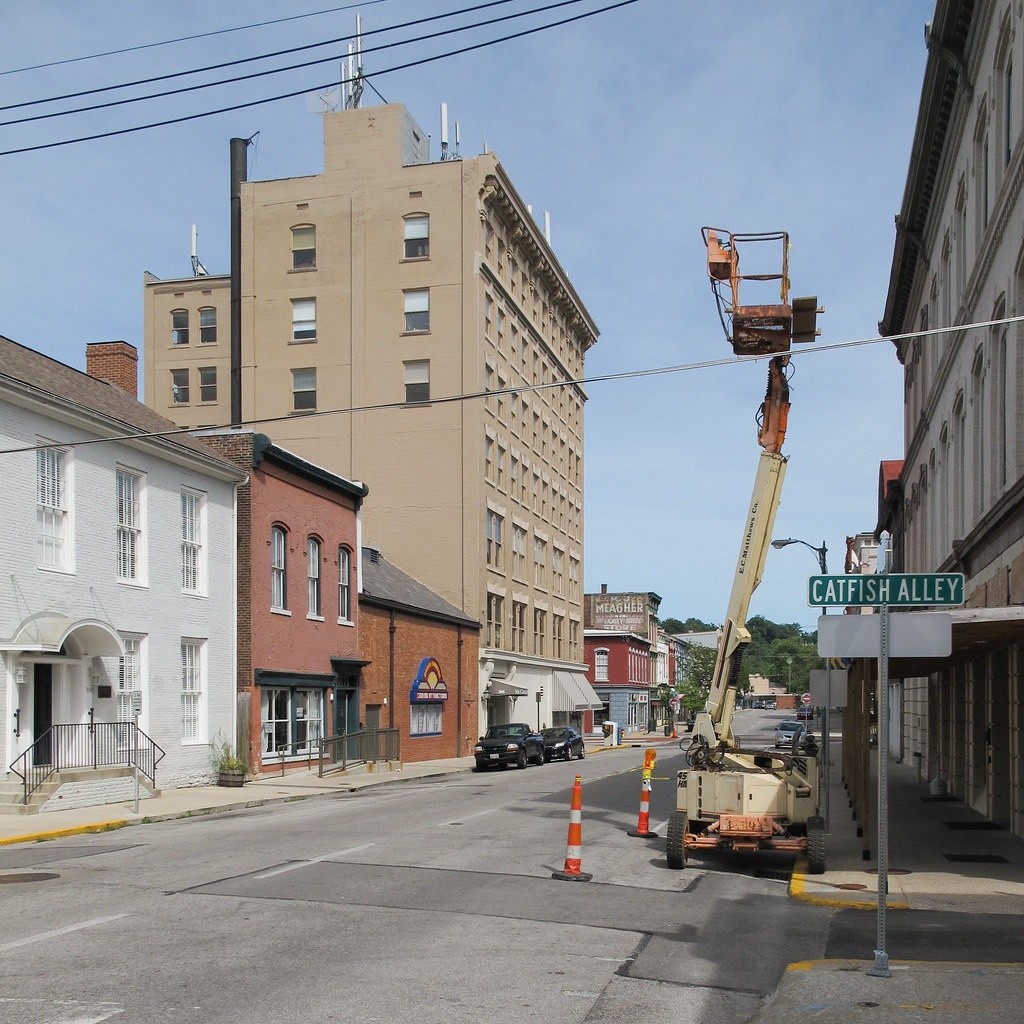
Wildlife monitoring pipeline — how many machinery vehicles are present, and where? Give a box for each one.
[666,226,828,875]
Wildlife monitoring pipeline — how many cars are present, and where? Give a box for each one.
[754,702,765,709]
[796,707,814,720]
[774,720,808,749]
[765,700,776,710]
[474,723,545,773]
[537,726,584,763]
[687,714,697,732]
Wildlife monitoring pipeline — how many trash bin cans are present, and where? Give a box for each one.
[664,725,671,736]
[687,720,695,732]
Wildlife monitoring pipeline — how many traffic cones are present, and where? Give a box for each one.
[672,728,677,738]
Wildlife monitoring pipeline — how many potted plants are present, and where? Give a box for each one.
[207,728,254,787]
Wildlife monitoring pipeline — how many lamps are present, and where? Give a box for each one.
[87,667,100,684]
[329,693,334,701]
[13,663,27,684]
[383,697,388,704]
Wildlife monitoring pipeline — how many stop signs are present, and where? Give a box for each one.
[801,694,811,704]
[670,698,678,706]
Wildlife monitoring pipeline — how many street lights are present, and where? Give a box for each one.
[772,539,828,764]
